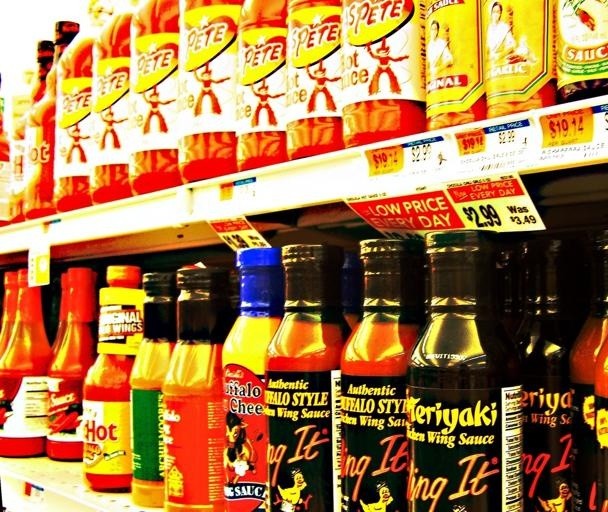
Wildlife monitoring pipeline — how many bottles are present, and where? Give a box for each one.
[0,0,608,512]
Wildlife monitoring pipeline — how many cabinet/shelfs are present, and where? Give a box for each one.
[0,93,608,512]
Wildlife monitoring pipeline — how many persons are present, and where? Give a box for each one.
[487,1,516,58]
[425,19,455,71]
